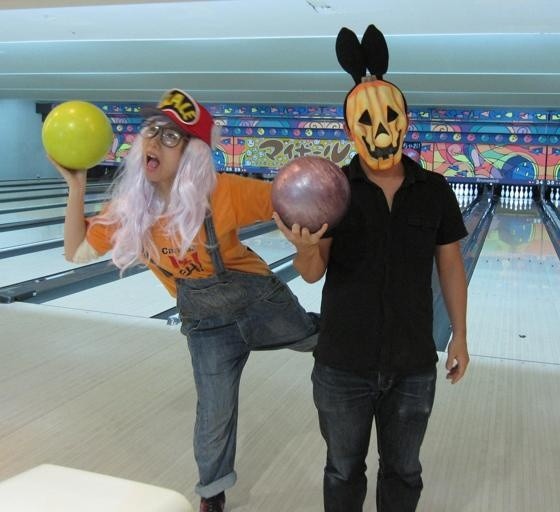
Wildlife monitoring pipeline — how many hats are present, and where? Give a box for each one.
[138,88,218,152]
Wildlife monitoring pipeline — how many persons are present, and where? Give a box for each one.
[272,21,471,512]
[47,88,320,512]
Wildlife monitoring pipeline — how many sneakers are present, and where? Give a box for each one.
[200,490,225,512]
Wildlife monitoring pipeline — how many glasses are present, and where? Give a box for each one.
[137,120,192,148]
